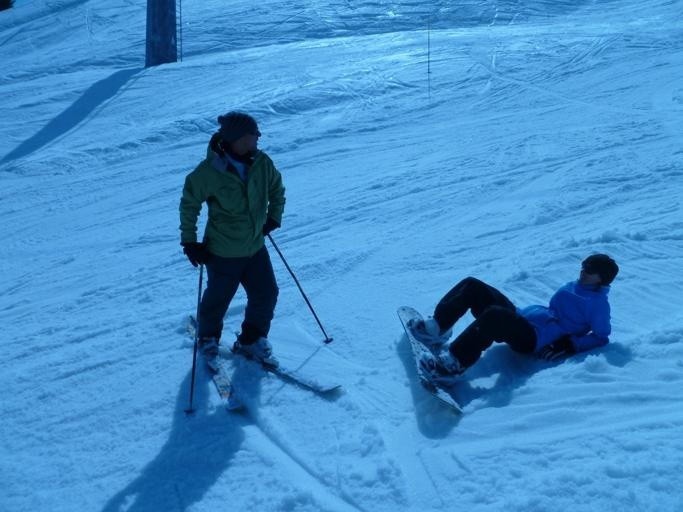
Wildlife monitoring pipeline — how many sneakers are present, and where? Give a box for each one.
[420,351,465,388]
[198,336,219,357]
[235,335,279,369]
[406,318,453,351]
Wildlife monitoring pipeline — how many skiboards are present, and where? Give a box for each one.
[188,314,341,411]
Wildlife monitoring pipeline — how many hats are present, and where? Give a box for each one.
[217,111,257,144]
[580,253,618,285]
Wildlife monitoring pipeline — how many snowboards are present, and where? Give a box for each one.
[396,306,464,415]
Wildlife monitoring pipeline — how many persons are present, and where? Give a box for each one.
[409,254,619,388]
[179,111,286,368]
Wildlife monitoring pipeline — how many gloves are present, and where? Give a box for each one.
[538,342,570,362]
[182,242,211,267]
[262,219,279,236]
